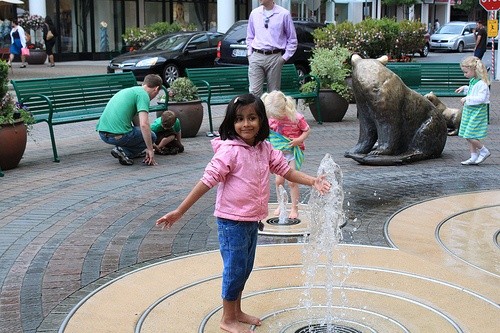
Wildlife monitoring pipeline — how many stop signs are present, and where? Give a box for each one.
[479,0,500,11]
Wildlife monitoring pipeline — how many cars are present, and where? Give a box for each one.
[107,31,224,88]
[372,27,430,58]
[430,21,498,53]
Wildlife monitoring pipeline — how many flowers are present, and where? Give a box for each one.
[18,15,47,49]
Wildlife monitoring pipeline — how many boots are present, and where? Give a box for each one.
[461,152,479,165]
[475,145,491,164]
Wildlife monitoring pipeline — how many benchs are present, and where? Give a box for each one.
[185,64,323,138]
[384,60,491,124]
[8,71,169,162]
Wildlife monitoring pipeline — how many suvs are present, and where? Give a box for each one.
[213,18,328,92]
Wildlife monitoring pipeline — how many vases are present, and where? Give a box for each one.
[27,49,47,65]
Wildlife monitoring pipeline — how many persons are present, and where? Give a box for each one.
[434,19,440,34]
[95,73,162,168]
[6,19,28,69]
[40,15,57,67]
[472,20,489,61]
[147,110,185,156]
[252,89,310,221]
[453,56,492,166]
[174,2,190,29]
[245,0,299,101]
[153,95,331,333]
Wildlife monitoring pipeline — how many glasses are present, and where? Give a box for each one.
[264,17,269,28]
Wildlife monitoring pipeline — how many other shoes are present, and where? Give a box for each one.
[110,147,134,165]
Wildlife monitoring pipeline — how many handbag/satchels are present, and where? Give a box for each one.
[21,46,31,57]
[45,22,54,41]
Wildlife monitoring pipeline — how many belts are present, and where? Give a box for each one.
[252,47,281,55]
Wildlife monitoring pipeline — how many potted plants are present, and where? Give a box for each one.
[0,59,35,172]
[154,78,204,138]
[297,46,354,122]
